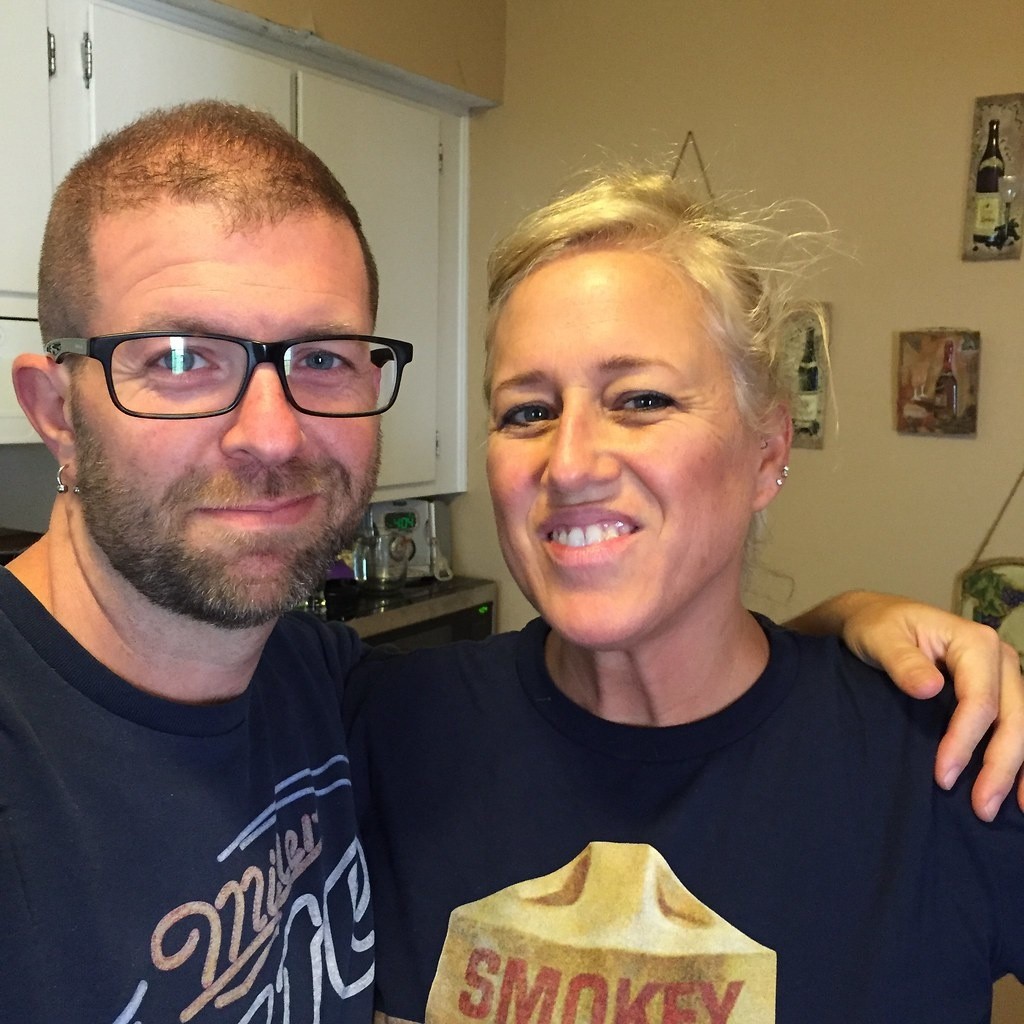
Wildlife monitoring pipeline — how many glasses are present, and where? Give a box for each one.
[42,331,416,419]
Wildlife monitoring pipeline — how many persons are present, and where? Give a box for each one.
[353,176,1024,1024]
[0,103,1024,1024]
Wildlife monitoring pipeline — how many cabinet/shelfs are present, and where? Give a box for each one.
[0,1,468,506]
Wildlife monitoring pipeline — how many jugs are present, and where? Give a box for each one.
[353,533,409,597]
